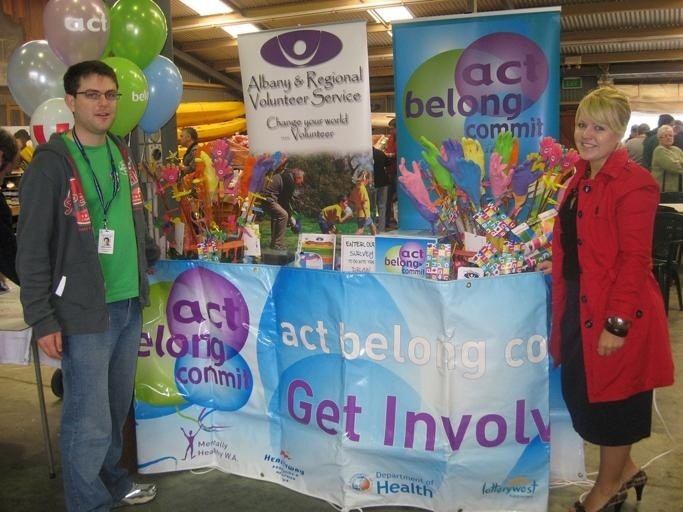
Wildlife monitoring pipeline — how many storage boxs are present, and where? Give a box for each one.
[371,226,460,283]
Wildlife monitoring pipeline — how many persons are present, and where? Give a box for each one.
[372,146,393,233]
[261,168,304,251]
[14,60,160,512]
[350,170,378,235]
[318,195,350,234]
[623,114,683,193]
[102,237,110,247]
[534,85,674,512]
[178,127,199,180]
[0,129,20,286]
[384,119,397,230]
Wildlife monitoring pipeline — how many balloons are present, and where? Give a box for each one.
[7,0,184,148]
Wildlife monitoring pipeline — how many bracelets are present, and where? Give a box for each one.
[605,322,629,337]
[390,153,392,158]
[182,165,184,170]
[608,316,631,329]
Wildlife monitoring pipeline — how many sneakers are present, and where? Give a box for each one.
[111,481,157,509]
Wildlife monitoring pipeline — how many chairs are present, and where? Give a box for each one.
[645,210,682,316]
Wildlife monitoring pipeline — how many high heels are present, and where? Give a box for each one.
[574,467,647,512]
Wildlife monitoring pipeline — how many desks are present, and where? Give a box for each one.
[0,284,58,481]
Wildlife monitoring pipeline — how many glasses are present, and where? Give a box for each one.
[70,88,122,101]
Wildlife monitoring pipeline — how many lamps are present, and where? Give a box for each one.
[177,0,267,38]
